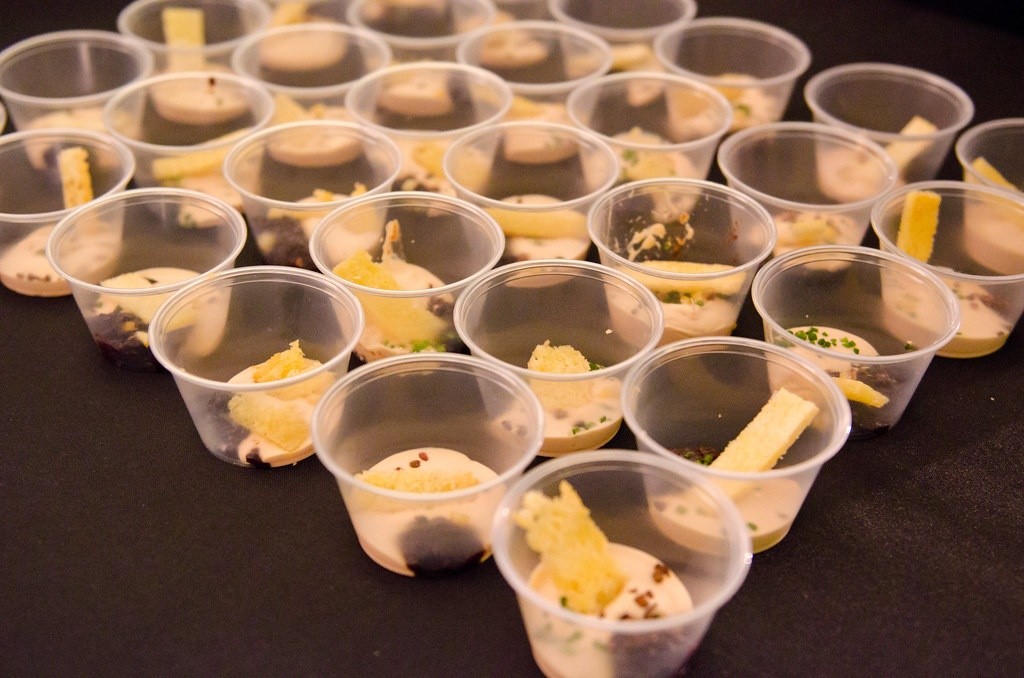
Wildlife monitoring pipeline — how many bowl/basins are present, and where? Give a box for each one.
[488,448,753,677]
[752,245,962,435]
[619,336,852,553]
[308,356,544,583]
[0,0,1023,370]
[455,260,663,459]
[148,264,360,465]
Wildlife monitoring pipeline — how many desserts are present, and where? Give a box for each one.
[0,0,1024,678]
